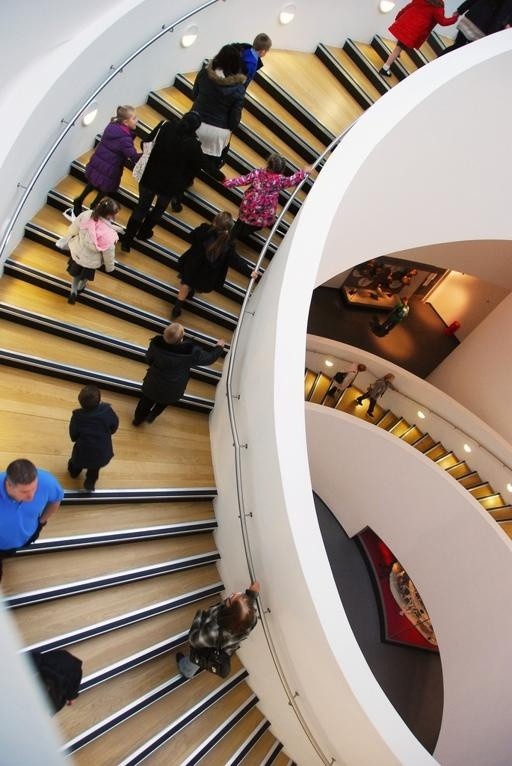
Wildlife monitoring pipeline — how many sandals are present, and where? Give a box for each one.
[380,68,391,77]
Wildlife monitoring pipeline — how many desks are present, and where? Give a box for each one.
[343,286,402,311]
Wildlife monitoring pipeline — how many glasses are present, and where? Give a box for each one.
[227,594,234,607]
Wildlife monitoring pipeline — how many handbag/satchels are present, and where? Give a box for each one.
[132,142,154,184]
[333,373,351,388]
[190,647,230,678]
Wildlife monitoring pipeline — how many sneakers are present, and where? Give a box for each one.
[68,293,77,304]
[77,288,84,294]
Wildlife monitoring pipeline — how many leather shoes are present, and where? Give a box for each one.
[176,653,184,662]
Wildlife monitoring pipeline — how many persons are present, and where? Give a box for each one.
[355,373,395,418]
[437,0,512,58]
[379,297,409,335]
[24,649,83,719]
[73,107,143,217]
[56,196,119,305]
[168,211,261,318]
[130,321,227,428]
[177,583,260,684]
[0,459,64,582]
[120,32,272,253]
[326,364,366,396]
[68,386,119,491]
[380,0,459,77]
[359,269,415,293]
[223,154,309,238]
